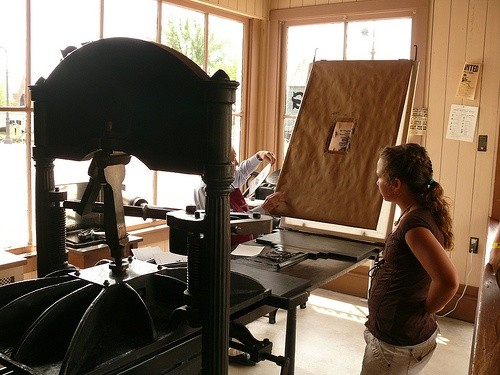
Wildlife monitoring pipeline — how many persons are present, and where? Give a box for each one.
[192,146,283,246]
[359,143,461,375]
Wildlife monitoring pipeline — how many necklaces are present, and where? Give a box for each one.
[393,198,418,226]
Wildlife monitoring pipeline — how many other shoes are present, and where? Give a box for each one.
[229,354,256,366]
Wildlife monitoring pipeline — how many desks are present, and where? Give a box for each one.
[230,224,381,375]
[67,232,144,270]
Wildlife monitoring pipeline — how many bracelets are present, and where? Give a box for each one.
[255,153,263,162]
